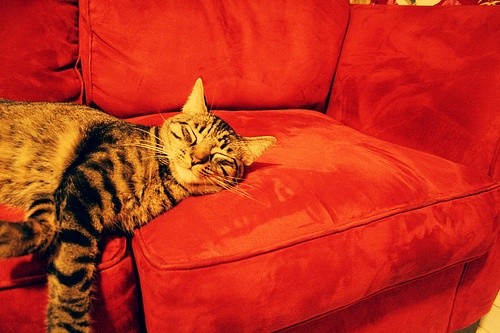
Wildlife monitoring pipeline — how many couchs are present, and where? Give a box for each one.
[1,0,500,332]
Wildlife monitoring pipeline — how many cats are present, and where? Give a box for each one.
[0,77,278,333]
